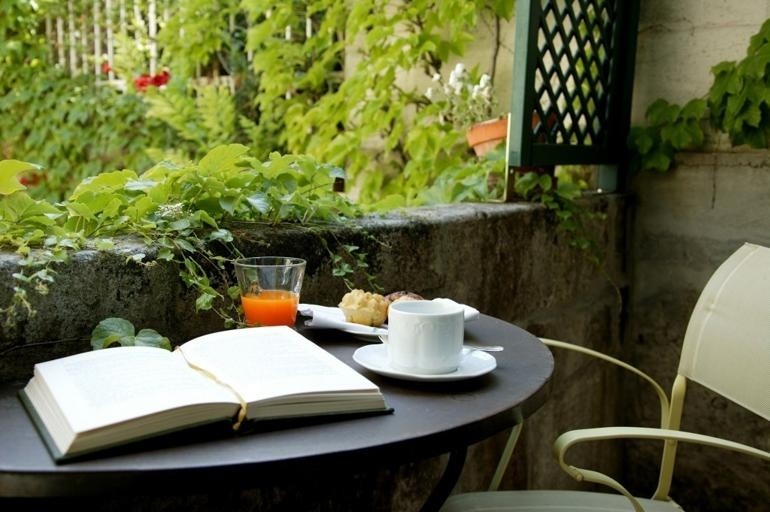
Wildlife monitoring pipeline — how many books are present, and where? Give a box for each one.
[15,322,396,466]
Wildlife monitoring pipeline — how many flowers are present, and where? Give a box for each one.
[424,60,500,135]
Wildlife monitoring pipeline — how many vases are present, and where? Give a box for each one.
[465,118,509,162]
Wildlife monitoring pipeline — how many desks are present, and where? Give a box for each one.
[1,306,557,510]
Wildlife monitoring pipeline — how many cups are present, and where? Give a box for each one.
[387,298,466,377]
[233,254,308,328]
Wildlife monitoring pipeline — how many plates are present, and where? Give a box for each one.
[352,343,498,384]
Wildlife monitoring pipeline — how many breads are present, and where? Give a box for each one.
[338,289,389,326]
[385,291,425,301]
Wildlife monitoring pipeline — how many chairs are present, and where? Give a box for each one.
[438,226,770,512]
